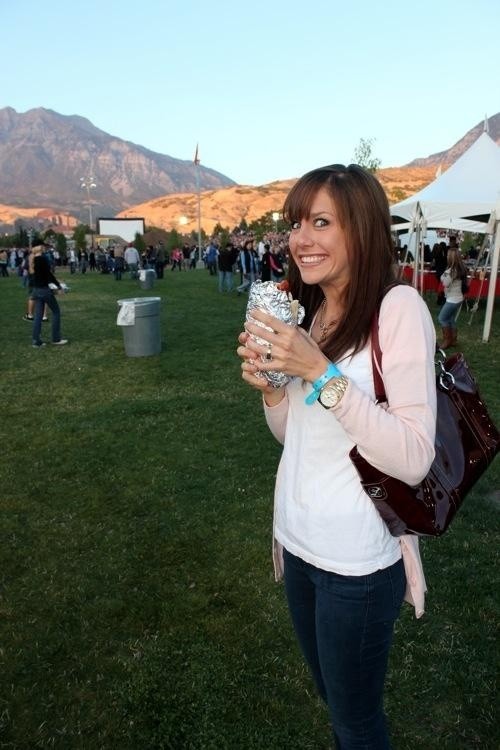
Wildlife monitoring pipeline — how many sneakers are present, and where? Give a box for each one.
[22,312,34,322]
[52,339,68,345]
[33,342,47,348]
[41,316,48,322]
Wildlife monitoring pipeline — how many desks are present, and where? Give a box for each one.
[396,254,500,308]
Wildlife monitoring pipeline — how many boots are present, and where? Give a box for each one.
[440,326,458,349]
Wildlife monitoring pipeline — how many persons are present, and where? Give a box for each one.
[0,238,198,282]
[237,163,437,749]
[201,227,292,295]
[393,240,490,279]
[22,239,50,323]
[436,246,470,351]
[29,238,69,350]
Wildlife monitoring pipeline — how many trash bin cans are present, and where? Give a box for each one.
[117,297,163,359]
[137,269,157,290]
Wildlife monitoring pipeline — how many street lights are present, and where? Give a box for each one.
[79,176,99,228]
[273,212,280,233]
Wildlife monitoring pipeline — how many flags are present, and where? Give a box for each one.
[191,147,202,167]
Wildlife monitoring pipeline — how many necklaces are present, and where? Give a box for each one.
[315,292,346,347]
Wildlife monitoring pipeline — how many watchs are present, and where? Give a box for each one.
[317,375,349,410]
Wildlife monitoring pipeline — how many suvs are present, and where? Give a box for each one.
[397,225,451,265]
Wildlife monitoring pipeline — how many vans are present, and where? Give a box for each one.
[96,235,127,248]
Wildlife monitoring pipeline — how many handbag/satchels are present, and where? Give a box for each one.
[348,282,500,539]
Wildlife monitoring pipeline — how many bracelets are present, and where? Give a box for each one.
[304,364,342,406]
